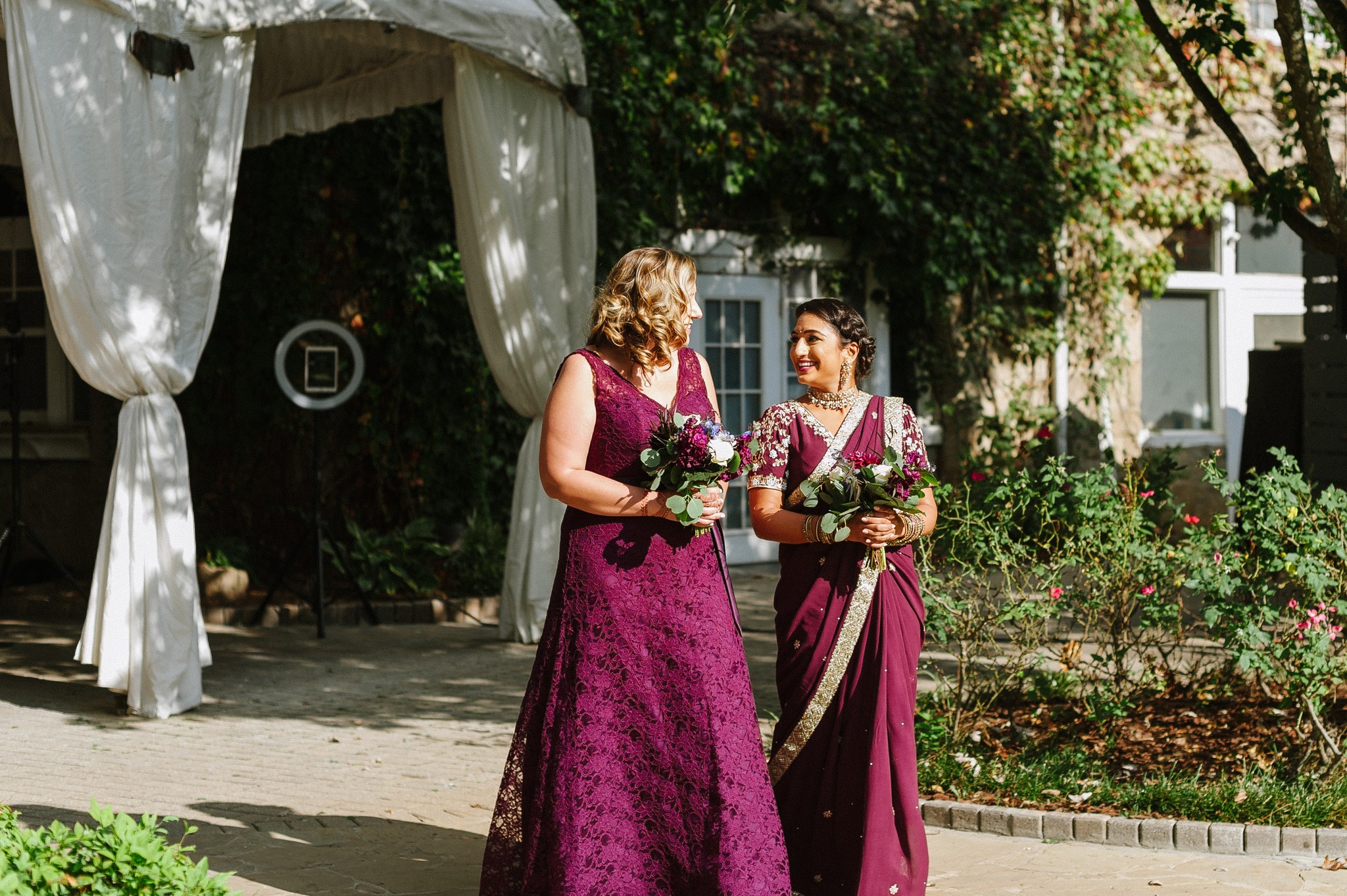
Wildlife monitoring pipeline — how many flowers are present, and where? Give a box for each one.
[637,400,765,531]
[798,417,942,572]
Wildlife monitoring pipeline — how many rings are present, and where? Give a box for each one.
[716,507,719,513]
[863,510,874,517]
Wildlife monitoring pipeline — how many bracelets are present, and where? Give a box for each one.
[885,512,925,546]
[802,514,848,545]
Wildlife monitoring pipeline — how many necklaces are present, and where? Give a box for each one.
[806,384,857,410]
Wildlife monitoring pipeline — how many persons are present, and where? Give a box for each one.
[474,245,792,895]
[744,294,940,896]
